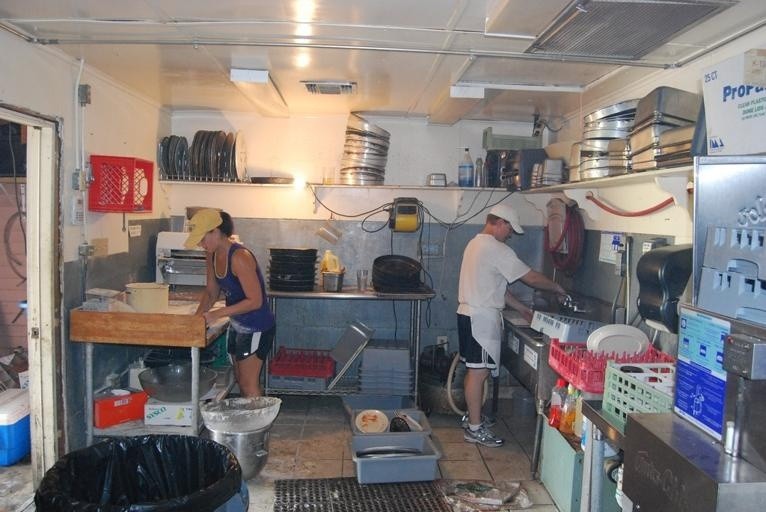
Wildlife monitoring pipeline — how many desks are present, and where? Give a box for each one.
[580,399,625,512]
[265,287,436,409]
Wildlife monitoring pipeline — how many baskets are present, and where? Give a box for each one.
[269,345,337,380]
[528,311,611,344]
[547,338,677,395]
[600,356,677,427]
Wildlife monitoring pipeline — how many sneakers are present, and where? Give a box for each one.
[463,426,505,448]
[461,411,499,431]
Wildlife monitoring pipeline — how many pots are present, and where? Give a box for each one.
[205,427,273,483]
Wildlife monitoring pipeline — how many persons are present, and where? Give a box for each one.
[183,208,276,431]
[457,204,570,448]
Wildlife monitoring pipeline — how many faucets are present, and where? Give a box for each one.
[560,292,586,315]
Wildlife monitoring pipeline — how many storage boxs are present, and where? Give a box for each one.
[341,395,420,417]
[0,388,31,466]
[700,49,766,156]
[347,433,443,484]
[94,387,148,429]
[352,410,433,437]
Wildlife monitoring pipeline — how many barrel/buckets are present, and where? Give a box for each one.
[124,281,170,308]
[320,249,341,286]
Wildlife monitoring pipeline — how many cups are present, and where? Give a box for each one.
[355,268,369,290]
[321,271,343,292]
[323,166,335,184]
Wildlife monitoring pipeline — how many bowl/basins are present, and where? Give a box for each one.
[199,395,281,432]
[137,363,218,404]
[339,111,390,187]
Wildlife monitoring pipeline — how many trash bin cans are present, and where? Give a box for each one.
[34,434,249,512]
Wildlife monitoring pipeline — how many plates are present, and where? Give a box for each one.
[585,324,650,358]
[398,410,424,432]
[159,128,245,181]
[249,175,294,184]
[265,246,319,292]
[356,444,421,459]
[390,417,411,432]
[354,409,387,434]
[371,255,423,294]
[579,99,638,180]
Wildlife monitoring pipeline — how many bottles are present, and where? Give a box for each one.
[547,378,587,452]
[457,147,474,186]
[475,156,483,186]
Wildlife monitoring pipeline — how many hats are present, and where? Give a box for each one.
[183,208,225,249]
[488,205,526,235]
